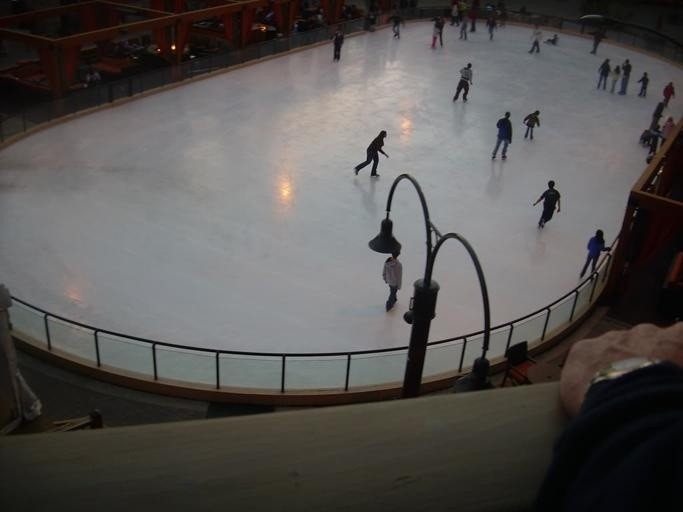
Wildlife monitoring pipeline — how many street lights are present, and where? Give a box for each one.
[367,171,497,398]
[575,14,683,56]
